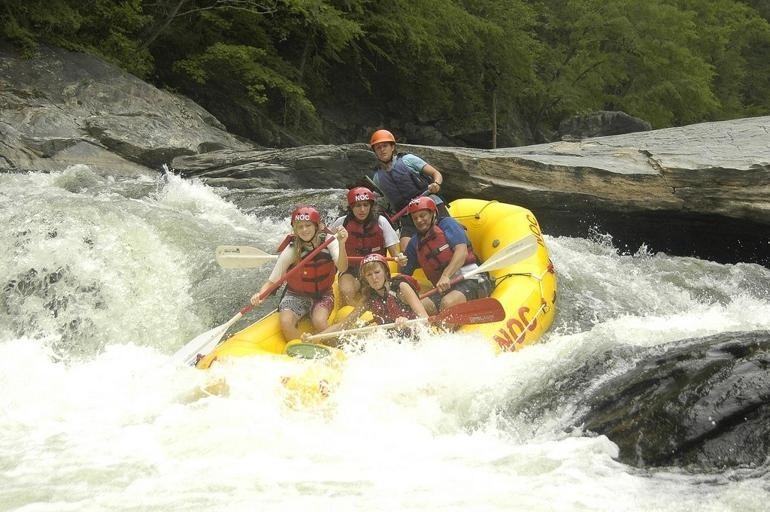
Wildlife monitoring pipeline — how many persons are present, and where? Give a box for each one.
[249,206,349,343]
[330,184,409,309]
[299,253,430,349]
[369,128,468,254]
[398,195,496,318]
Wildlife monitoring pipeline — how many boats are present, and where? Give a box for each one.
[193,198,559,412]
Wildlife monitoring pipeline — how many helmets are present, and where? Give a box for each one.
[348,187,374,206]
[291,208,319,227]
[370,130,395,146]
[359,254,389,272]
[408,197,437,213]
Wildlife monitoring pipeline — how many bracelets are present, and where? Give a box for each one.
[432,179,441,187]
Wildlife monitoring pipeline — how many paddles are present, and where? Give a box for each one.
[173,229,347,365]
[217,246,401,271]
[416,236,537,300]
[308,298,506,340]
